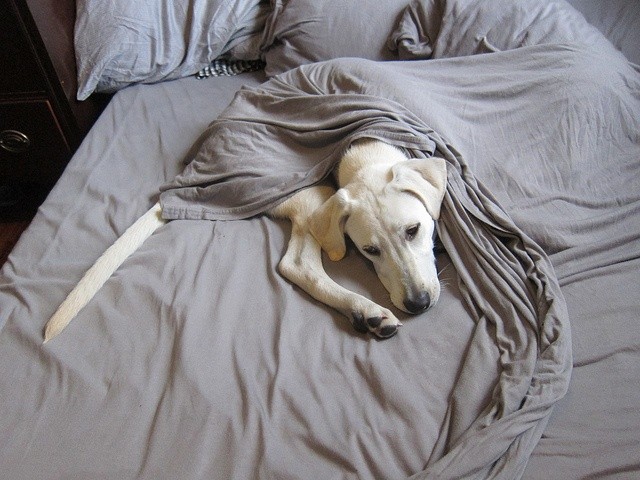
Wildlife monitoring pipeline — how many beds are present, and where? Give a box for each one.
[0,1,638,479]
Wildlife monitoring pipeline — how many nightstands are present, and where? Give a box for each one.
[2,3,107,228]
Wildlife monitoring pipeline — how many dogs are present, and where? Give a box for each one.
[41,137,449,342]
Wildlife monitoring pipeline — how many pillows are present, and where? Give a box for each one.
[277,2,433,70]
[73,3,294,104]
